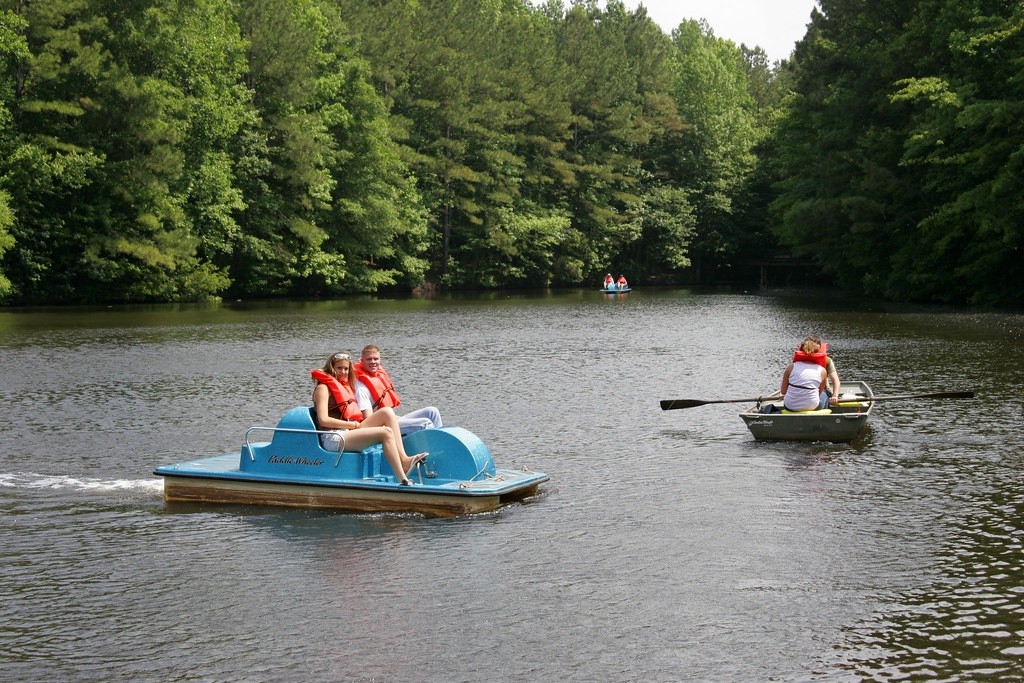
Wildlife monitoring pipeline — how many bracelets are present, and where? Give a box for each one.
[355,421,356,428]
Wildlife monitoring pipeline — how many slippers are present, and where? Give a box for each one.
[405,452,429,477]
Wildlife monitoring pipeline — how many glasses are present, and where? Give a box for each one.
[333,353,350,360]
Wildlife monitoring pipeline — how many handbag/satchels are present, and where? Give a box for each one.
[759,404,781,414]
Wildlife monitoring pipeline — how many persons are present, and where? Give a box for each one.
[604,273,614,289]
[781,335,840,413]
[352,345,443,436]
[617,275,627,290]
[312,352,429,486]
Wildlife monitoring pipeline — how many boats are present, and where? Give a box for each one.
[153,407,550,519]
[738,380,875,442]
[600,284,632,293]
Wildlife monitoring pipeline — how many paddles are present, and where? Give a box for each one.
[659,391,975,410]
